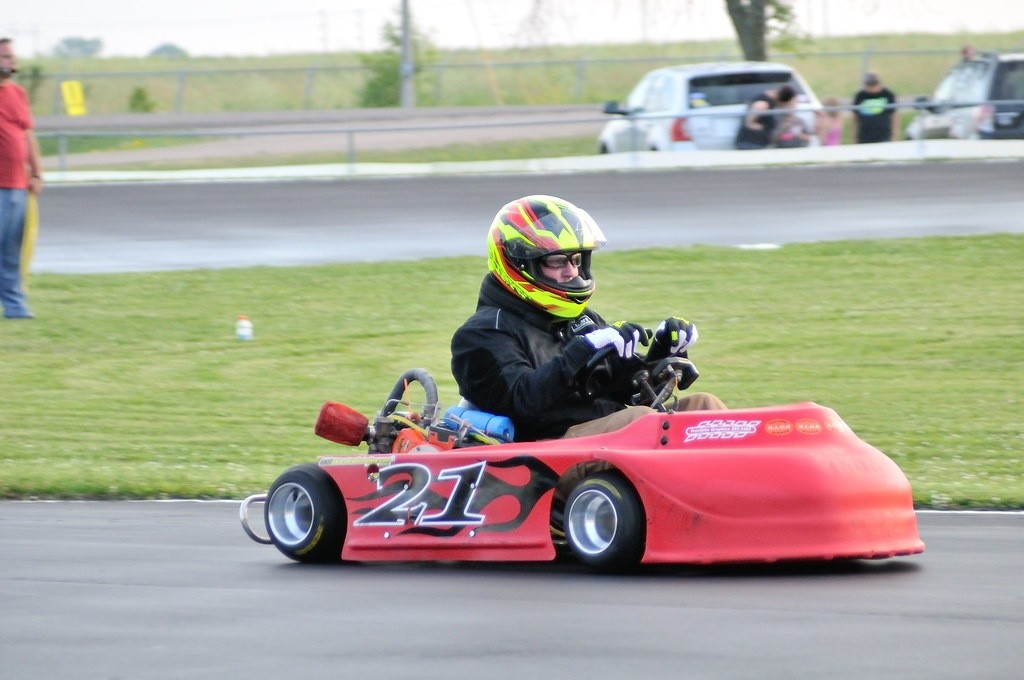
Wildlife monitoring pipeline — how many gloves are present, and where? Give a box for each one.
[585,321,650,358]
[656,316,698,354]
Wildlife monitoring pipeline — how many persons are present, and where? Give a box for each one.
[850,72,900,142]
[0,37,42,318]
[778,99,809,148]
[451,194,728,443]
[816,96,844,145]
[735,86,794,148]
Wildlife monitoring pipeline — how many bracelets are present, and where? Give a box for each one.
[33,176,40,178]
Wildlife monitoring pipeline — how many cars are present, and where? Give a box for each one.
[906,48,1024,140]
[599,59,825,155]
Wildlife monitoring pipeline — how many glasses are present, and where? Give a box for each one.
[539,253,582,268]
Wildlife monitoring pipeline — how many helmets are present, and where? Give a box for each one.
[488,194,598,319]
